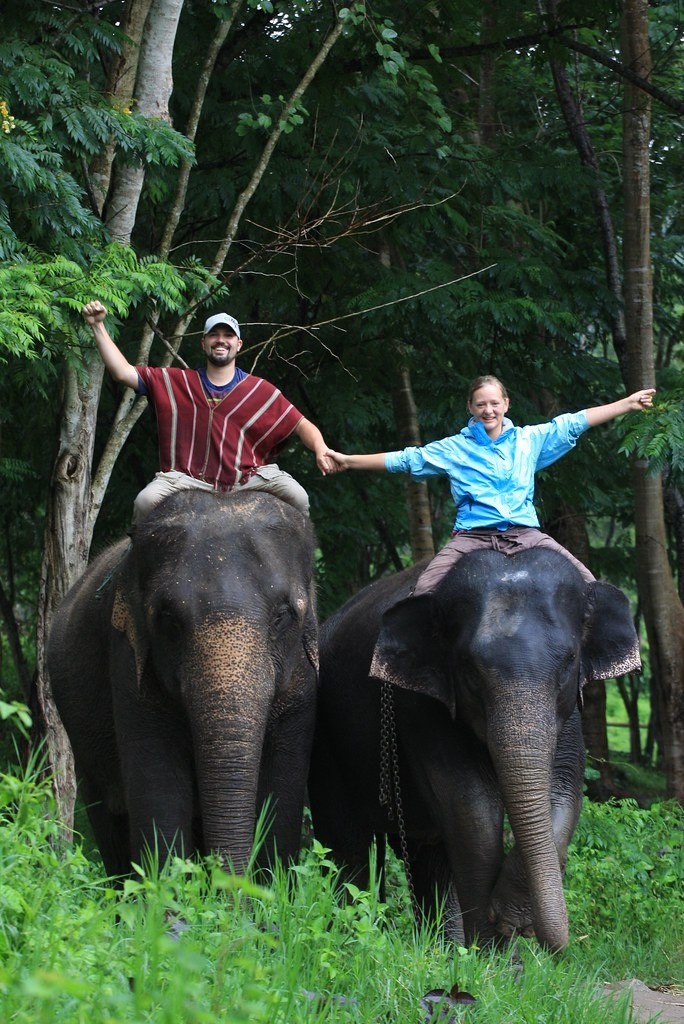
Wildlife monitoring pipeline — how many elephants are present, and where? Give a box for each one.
[44,485,322,930]
[314,543,645,962]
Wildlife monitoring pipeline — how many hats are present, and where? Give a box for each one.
[203,313,240,340]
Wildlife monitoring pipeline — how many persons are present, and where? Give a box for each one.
[77,299,340,528]
[322,374,658,596]
